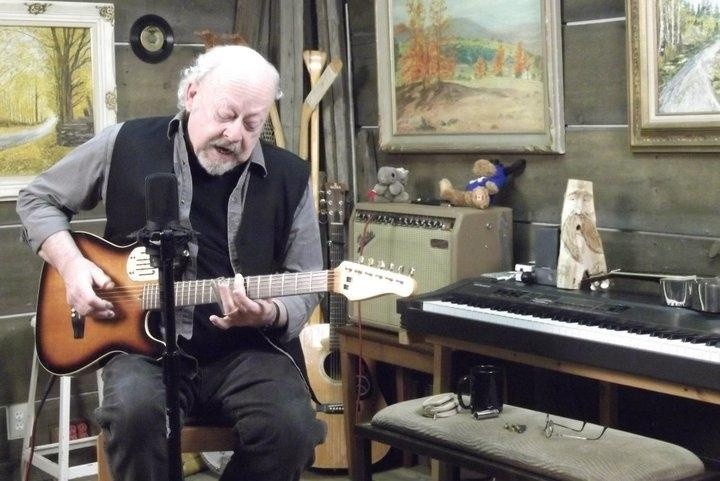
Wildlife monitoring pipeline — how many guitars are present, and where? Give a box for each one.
[33,230,419,377]
[297,179,393,475]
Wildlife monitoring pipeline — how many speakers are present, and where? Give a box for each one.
[346,199,514,337]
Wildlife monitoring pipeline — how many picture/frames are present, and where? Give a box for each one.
[625,0,720,153]
[0,3,119,203]
[375,1,567,156]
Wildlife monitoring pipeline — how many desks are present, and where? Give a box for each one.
[334,323,454,481]
[422,333,720,481]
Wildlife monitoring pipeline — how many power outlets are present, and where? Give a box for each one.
[8,404,27,439]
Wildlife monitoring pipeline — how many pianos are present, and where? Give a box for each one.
[396,275,720,399]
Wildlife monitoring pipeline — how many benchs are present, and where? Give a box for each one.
[356,391,706,481]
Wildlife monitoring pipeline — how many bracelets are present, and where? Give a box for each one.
[263,300,280,335]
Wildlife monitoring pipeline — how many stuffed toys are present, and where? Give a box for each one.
[437,155,527,210]
[369,165,411,203]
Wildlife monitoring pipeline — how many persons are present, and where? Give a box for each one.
[14,41,333,480]
[557,178,607,288]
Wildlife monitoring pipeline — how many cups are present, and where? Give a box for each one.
[456,359,506,420]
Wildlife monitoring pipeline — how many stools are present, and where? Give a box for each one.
[20,317,104,481]
[94,424,316,481]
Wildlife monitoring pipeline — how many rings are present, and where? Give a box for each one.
[224,307,240,317]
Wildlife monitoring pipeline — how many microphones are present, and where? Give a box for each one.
[143,172,179,267]
